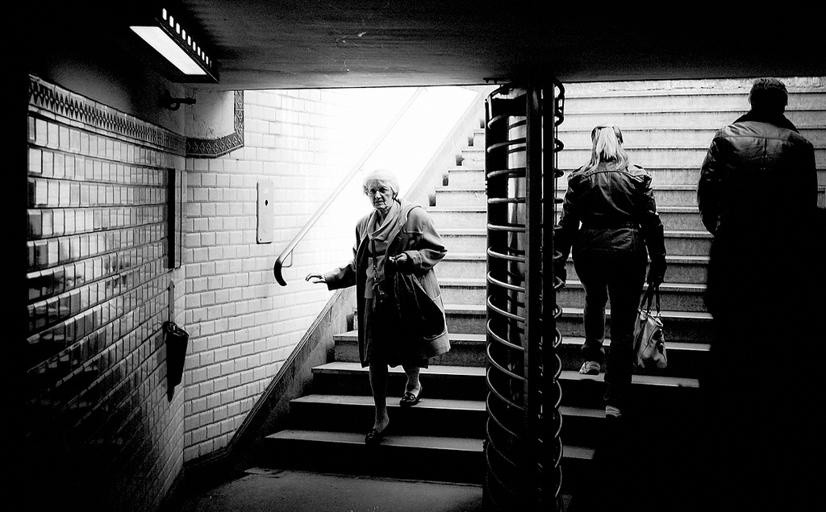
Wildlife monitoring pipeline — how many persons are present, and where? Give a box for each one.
[696,76,819,237]
[553,123,670,420]
[302,167,453,448]
[575,193,825,510]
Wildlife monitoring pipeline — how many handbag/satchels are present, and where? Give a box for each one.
[632,281,668,372]
[374,259,424,327]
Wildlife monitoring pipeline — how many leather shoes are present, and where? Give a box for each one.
[399,384,423,406]
[365,419,391,444]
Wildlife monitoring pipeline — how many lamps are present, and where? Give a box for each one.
[124,8,219,85]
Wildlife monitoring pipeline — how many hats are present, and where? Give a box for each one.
[750,78,789,96]
[590,124,624,142]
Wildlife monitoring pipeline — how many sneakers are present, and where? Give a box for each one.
[578,360,601,376]
[605,404,622,419]
